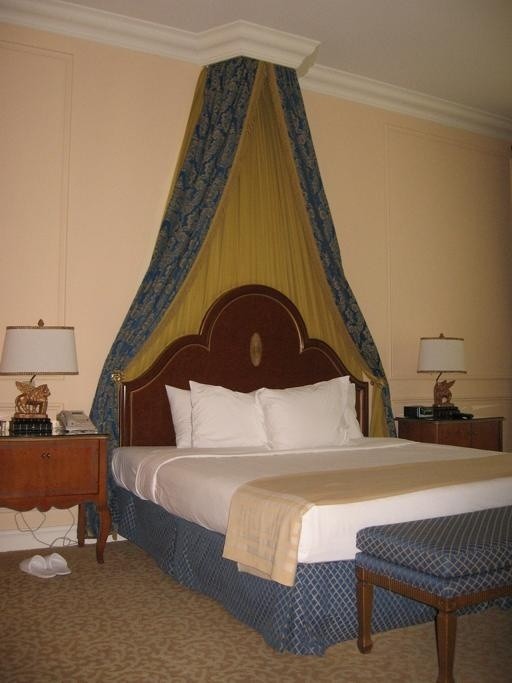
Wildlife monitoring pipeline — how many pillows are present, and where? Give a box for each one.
[166,375,363,453]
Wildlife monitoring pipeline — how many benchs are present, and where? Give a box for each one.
[354,506,511,683]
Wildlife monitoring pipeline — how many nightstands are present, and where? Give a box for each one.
[1,434,111,565]
[395,417,506,452]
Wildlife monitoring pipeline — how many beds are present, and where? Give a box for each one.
[112,284,510,655]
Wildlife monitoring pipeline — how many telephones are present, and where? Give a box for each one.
[56,410,99,436]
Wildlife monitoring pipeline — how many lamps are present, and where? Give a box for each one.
[1,318,78,437]
[417,333,468,420]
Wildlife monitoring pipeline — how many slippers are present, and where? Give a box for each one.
[18,555,57,579]
[44,553,71,575]
